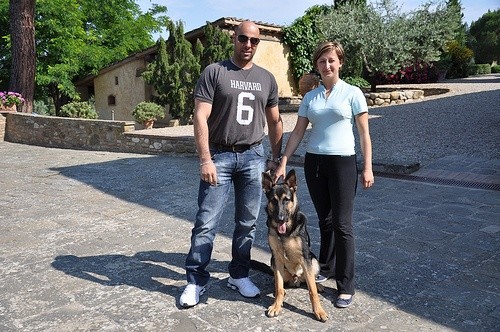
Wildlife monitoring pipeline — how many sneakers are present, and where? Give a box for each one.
[315,275,329,282]
[180,283,206,308]
[336,294,353,308]
[226,276,260,299]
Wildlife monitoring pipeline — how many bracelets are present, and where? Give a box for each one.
[198,160,215,167]
[272,155,283,163]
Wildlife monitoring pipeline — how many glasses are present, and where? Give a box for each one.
[236,33,260,45]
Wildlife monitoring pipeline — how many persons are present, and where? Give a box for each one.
[177,21,283,309]
[273,41,376,309]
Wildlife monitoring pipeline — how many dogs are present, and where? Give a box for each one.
[262,168,329,322]
